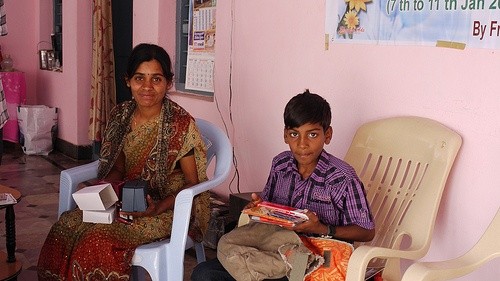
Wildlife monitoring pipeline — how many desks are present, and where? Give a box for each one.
[0,68,26,151]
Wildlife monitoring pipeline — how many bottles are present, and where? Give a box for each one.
[0,53,15,73]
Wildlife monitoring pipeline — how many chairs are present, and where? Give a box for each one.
[59,120,233,281]
[399,206,500,281]
[237,115,463,281]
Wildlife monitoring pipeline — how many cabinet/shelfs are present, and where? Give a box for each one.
[0,184,22,281]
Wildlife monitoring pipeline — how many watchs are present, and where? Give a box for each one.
[320,224,335,240]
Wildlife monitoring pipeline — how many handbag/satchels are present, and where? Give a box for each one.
[16,105,58,156]
[217,220,354,281]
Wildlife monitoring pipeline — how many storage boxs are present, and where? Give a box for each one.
[71,182,119,224]
[122,180,149,212]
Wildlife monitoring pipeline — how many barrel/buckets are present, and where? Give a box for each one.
[37,40,56,69]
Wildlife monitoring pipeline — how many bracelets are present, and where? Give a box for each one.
[170,193,176,199]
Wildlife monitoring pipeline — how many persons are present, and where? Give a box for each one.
[37,43,209,281]
[216,92,374,281]
